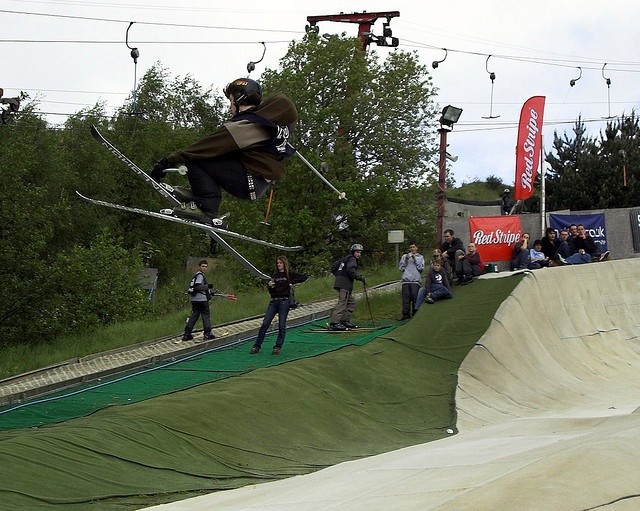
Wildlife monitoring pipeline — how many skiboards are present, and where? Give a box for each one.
[76,124,304,279]
[303,328,375,333]
[173,331,229,344]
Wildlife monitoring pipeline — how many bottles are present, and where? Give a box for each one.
[495,266,498,272]
[580,249,585,255]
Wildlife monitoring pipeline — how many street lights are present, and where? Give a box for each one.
[436,105,464,258]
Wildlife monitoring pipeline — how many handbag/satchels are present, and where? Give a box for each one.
[289,288,299,308]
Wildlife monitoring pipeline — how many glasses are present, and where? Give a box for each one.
[444,235,450,237]
[523,235,529,239]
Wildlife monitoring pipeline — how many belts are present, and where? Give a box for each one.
[248,174,257,201]
[270,297,288,300]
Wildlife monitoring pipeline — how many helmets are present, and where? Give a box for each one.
[433,248,442,256]
[350,243,363,251]
[227,77,262,105]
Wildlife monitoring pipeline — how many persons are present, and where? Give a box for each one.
[438,229,466,261]
[149,78,287,216]
[511,233,530,268]
[559,230,571,258]
[330,243,363,330]
[425,260,453,303]
[415,249,452,309]
[541,228,561,259]
[455,242,482,286]
[182,259,215,340]
[502,189,512,211]
[567,224,577,243]
[251,255,308,357]
[529,240,549,269]
[399,243,425,319]
[574,225,609,261]
[455,250,466,261]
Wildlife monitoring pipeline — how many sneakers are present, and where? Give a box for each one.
[251,345,260,353]
[555,252,567,265]
[173,200,217,225]
[204,331,215,339]
[272,345,281,353]
[461,275,474,285]
[329,322,346,330]
[183,332,193,340]
[514,267,519,270]
[425,294,435,303]
[455,275,464,285]
[172,184,194,203]
[340,320,355,327]
[598,249,609,260]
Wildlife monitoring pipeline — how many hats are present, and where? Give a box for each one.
[534,239,542,244]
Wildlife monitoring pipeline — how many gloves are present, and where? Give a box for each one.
[208,283,213,288]
[149,154,170,183]
